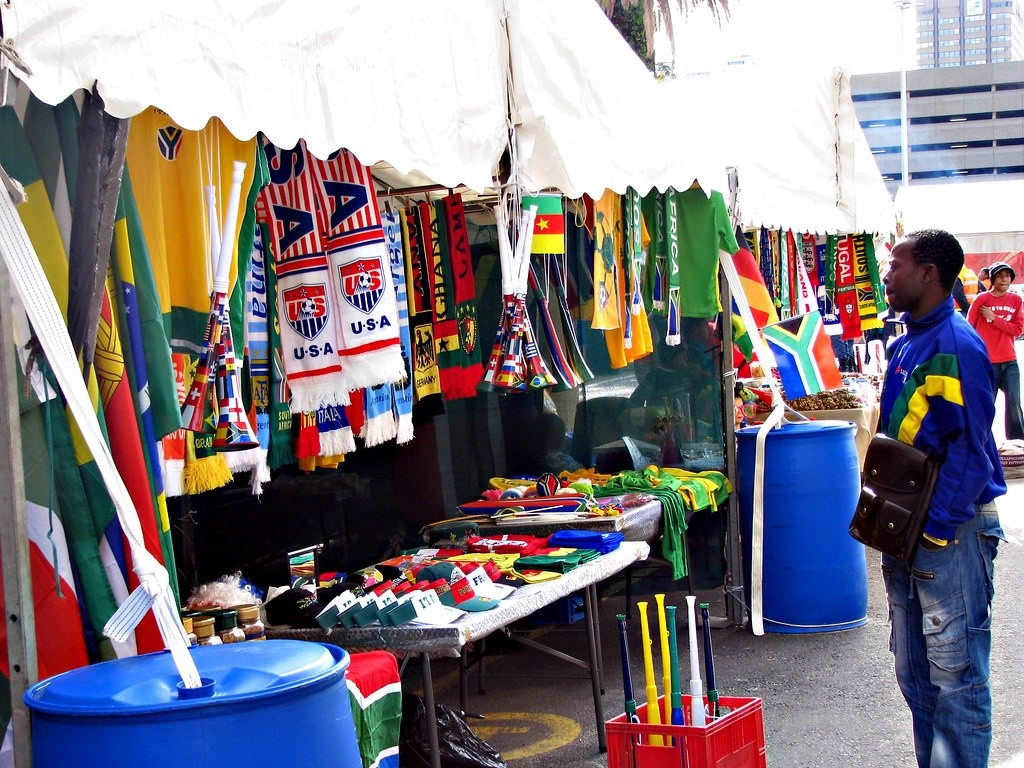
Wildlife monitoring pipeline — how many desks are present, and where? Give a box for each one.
[262,539,650,768]
[428,478,724,694]
[751,405,881,464]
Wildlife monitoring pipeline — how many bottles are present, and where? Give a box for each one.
[182,606,266,649]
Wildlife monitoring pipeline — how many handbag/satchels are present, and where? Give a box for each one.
[848,434,942,564]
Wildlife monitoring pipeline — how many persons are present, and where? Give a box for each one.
[875,230,1009,768]
[967,261,1024,440]
[977,268,992,295]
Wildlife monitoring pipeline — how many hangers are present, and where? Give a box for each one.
[387,186,394,211]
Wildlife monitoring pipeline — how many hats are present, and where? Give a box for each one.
[989,262,1016,286]
[264,559,524,631]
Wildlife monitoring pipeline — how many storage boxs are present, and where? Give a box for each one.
[606,694,766,768]
[527,590,585,626]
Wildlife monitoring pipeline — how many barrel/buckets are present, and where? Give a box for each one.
[23,638,363,768]
[733,419,869,632]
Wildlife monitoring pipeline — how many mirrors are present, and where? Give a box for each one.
[286,545,319,582]
